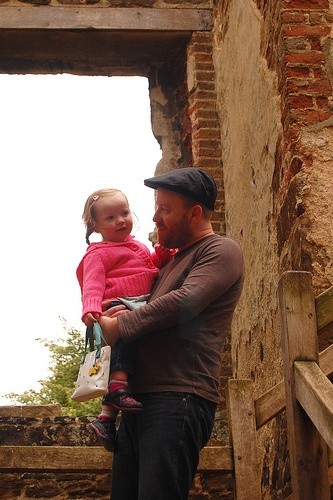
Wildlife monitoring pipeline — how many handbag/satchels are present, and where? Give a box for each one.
[71,322,111,403]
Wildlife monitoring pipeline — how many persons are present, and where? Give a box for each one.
[100,166,244,499]
[77,188,179,453]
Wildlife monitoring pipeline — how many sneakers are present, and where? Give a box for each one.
[102,388,144,413]
[89,419,116,452]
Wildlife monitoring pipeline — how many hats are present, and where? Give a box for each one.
[144,168,217,207]
